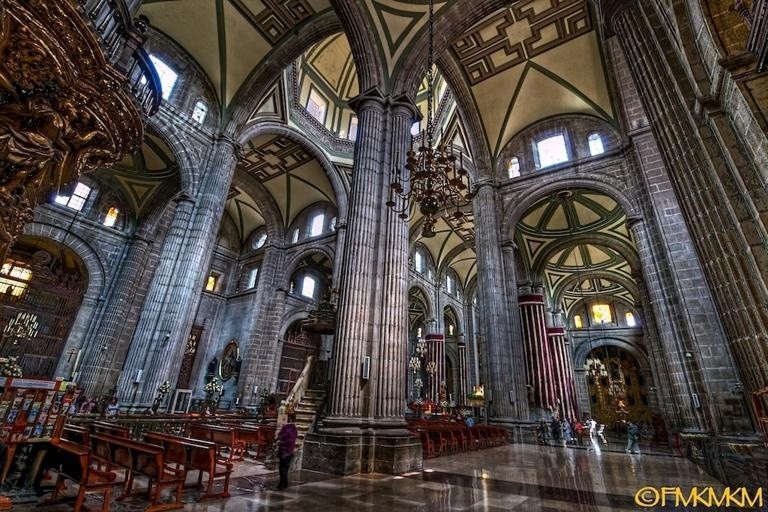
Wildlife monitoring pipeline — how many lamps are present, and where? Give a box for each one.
[159,380,171,393]
[0,312,42,357]
[0,356,25,378]
[409,338,437,389]
[202,376,221,394]
[385,0,476,237]
[556,190,609,383]
[184,333,197,358]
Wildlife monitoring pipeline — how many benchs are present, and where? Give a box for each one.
[46,406,513,512]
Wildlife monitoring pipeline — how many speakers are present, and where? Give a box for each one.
[692,394,700,409]
[72,371,82,383]
[362,356,371,380]
[134,368,143,384]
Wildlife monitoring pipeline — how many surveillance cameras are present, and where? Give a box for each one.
[165,333,171,340]
[101,343,107,350]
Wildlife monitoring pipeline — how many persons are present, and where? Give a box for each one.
[444,413,484,427]
[154,394,163,409]
[624,419,642,454]
[539,416,597,446]
[275,412,298,489]
[610,420,617,437]
